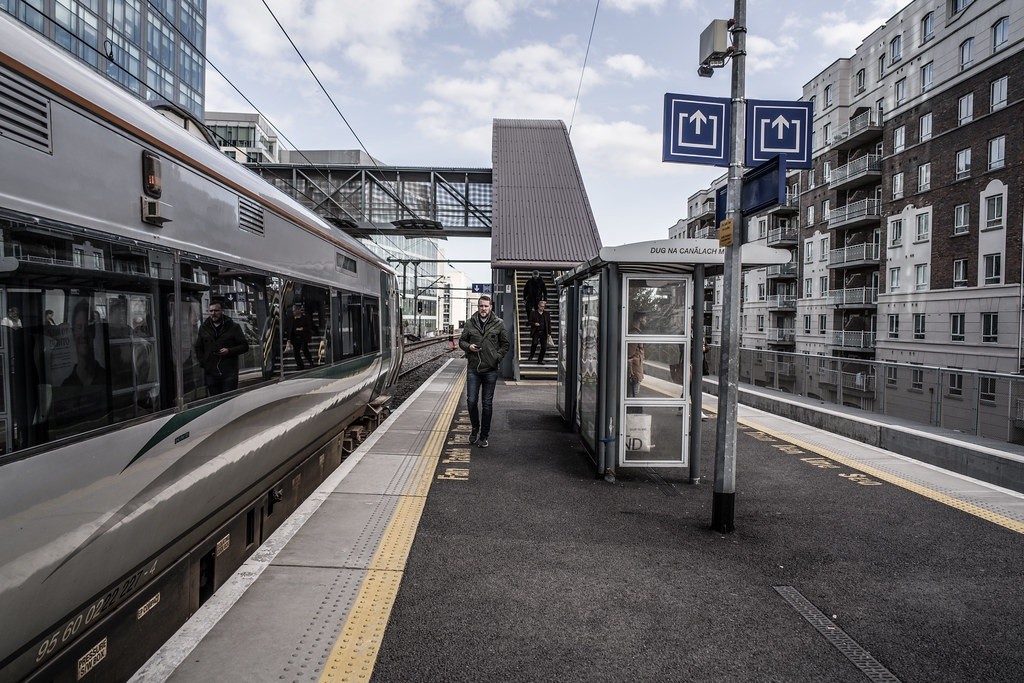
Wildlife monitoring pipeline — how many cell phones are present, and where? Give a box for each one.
[474,346,481,349]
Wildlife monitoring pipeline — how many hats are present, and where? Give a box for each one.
[532,270,539,277]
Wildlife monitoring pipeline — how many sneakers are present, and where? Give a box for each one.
[469,427,480,443]
[478,437,488,447]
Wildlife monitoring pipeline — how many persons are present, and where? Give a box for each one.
[528,301,551,366]
[459,296,510,448]
[677,318,710,418]
[523,270,547,327]
[2,279,326,451]
[627,311,655,448]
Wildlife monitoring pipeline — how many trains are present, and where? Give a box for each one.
[0,8,405,683]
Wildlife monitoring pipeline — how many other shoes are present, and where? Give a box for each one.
[528,358,531,361]
[700,411,709,420]
[537,362,545,365]
[678,410,683,417]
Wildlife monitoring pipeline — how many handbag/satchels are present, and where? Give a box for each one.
[669,363,694,385]
[283,340,294,353]
[547,334,555,347]
